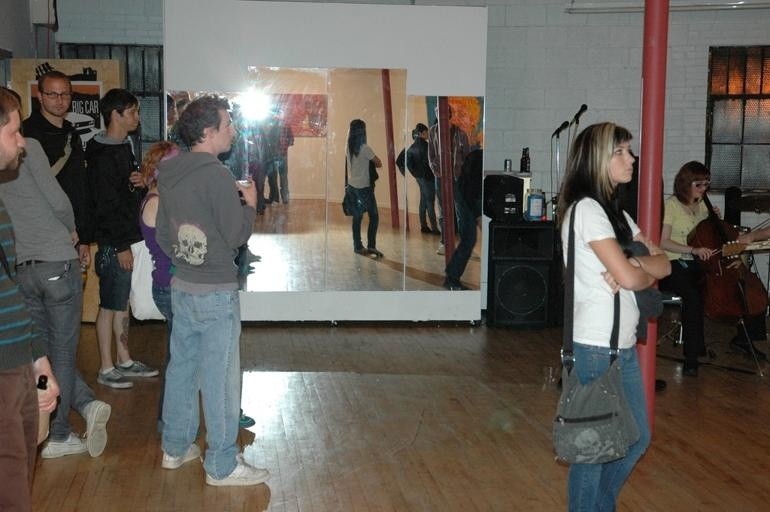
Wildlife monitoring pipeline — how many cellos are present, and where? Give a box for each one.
[687,190,768,324]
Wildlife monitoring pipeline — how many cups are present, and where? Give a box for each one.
[236,179,252,206]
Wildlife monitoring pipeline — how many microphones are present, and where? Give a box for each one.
[570,104,587,126]
[732,225,747,232]
[552,121,569,138]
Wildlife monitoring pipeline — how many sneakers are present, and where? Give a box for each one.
[729,336,766,359]
[369,247,383,256]
[206,456,270,487]
[95,368,134,389]
[82,401,111,458]
[681,355,699,376]
[163,444,201,470]
[353,245,368,254]
[116,363,160,379]
[40,431,87,459]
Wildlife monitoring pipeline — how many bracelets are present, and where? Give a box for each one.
[690,245,695,254]
[631,256,644,271]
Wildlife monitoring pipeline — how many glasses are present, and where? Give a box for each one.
[694,182,710,188]
[38,88,72,99]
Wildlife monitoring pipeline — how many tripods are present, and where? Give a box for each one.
[658,301,706,349]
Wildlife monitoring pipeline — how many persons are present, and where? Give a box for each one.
[735,225,769,244]
[168,91,293,276]
[659,156,769,378]
[620,147,668,392]
[554,123,675,511]
[0,85,112,459]
[397,101,483,291]
[79,85,160,391]
[19,71,90,296]
[140,140,256,433]
[344,119,385,258]
[1,86,50,510]
[159,93,271,486]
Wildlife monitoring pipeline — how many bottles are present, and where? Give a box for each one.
[519,147,530,173]
[525,188,558,223]
[80,251,87,291]
[133,160,139,173]
[36,374,50,390]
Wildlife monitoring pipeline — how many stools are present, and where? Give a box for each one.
[656,293,684,350]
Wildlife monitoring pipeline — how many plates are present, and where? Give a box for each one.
[516,172,531,177]
[502,171,514,175]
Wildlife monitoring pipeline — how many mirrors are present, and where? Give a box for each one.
[222,67,485,292]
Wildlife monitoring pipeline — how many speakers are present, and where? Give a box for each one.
[487,219,564,329]
[483,175,524,221]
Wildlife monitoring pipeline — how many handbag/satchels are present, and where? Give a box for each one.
[554,362,640,464]
[342,190,353,215]
